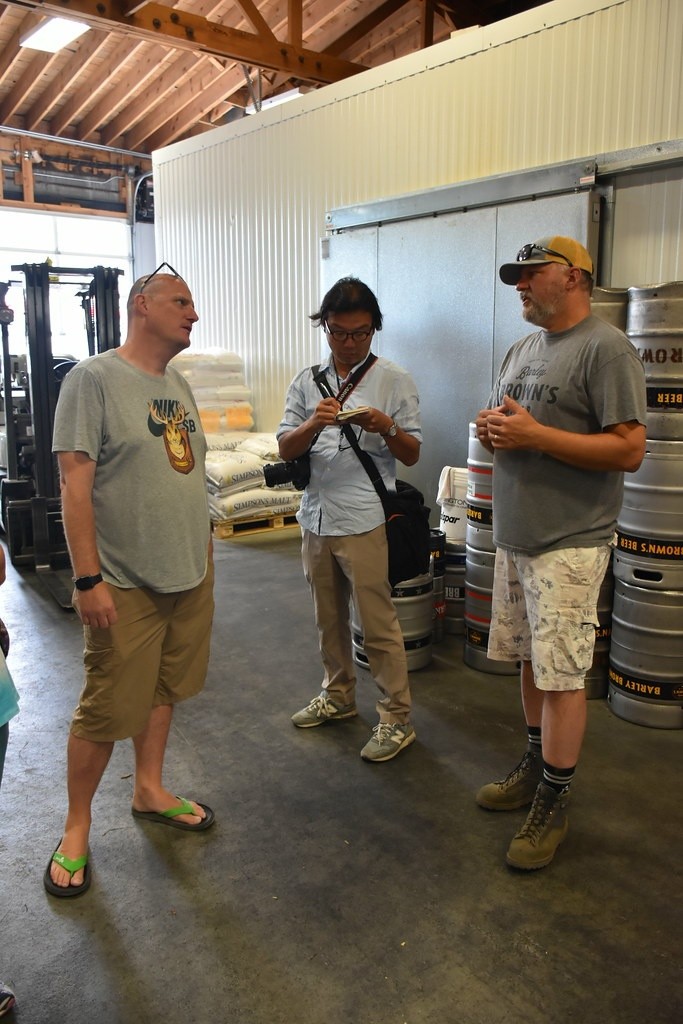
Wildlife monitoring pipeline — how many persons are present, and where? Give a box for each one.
[275,276,423,763]
[0,526,18,1020]
[42,263,216,898]
[475,235,648,870]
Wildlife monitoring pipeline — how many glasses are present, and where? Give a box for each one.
[324,319,373,342]
[515,245,573,268]
[137,262,179,294]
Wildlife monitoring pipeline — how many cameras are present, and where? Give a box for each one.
[263,452,310,491]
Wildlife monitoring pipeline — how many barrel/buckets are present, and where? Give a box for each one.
[353,278,683,728]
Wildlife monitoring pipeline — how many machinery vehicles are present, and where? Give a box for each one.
[0,257,129,609]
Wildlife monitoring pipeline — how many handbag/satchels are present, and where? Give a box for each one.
[385,479,431,586]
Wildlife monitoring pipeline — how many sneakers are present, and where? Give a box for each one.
[291,690,360,727]
[360,722,416,761]
[506,783,573,869]
[476,743,544,810]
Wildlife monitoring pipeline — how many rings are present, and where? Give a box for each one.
[493,434,498,441]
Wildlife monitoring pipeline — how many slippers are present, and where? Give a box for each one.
[132,796,213,830]
[44,835,91,897]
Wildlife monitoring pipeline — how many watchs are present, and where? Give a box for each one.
[70,574,102,594]
[379,421,399,439]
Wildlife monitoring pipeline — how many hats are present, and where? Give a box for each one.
[500,236,594,286]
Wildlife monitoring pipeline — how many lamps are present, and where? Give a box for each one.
[24,149,30,161]
[18,17,90,53]
[246,86,309,115]
